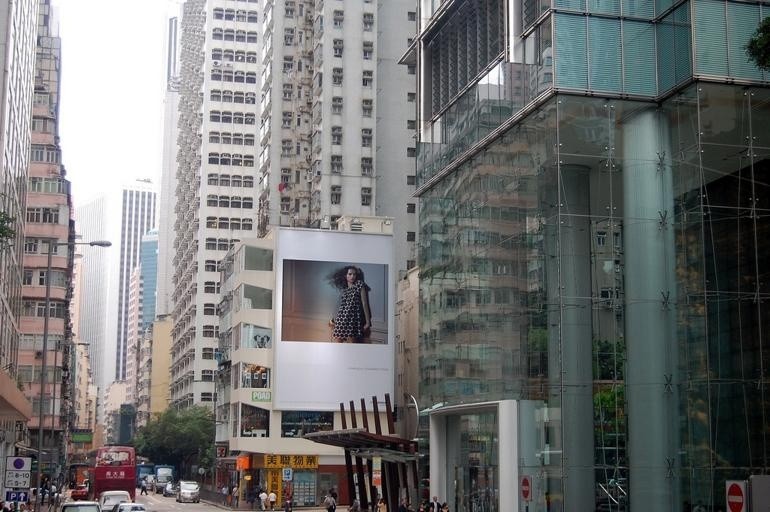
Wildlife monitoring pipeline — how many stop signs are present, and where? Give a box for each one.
[726,482,746,512]
[521,477,532,501]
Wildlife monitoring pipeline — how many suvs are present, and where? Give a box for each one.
[594,461,629,506]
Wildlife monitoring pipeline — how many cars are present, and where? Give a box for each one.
[47,445,200,512]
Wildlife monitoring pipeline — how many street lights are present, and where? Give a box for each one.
[35,239,112,511]
[49,341,91,487]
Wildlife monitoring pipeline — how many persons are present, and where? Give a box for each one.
[140,478,148,496]
[246,486,277,512]
[149,477,157,496]
[399,495,449,512]
[323,487,337,512]
[0,479,70,512]
[285,497,292,512]
[347,486,387,512]
[222,481,239,509]
[328,266,373,342]
[455,480,497,512]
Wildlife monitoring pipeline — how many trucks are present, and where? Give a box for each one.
[468,435,497,467]
